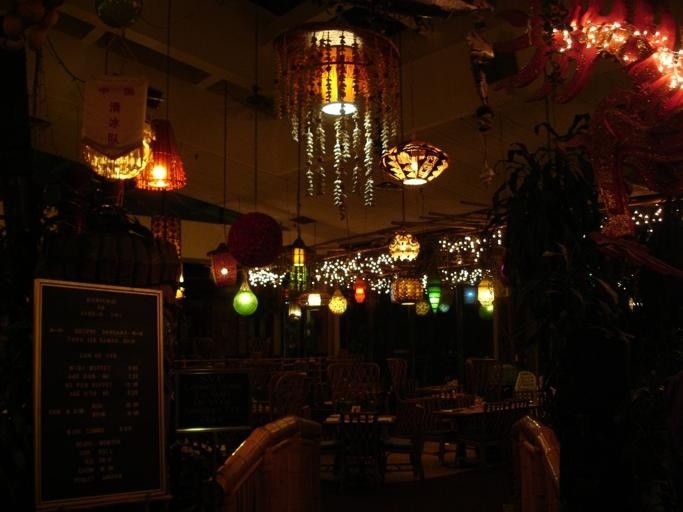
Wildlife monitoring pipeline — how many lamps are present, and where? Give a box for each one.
[70,62,190,309]
[521,1,682,243]
[271,2,454,201]
